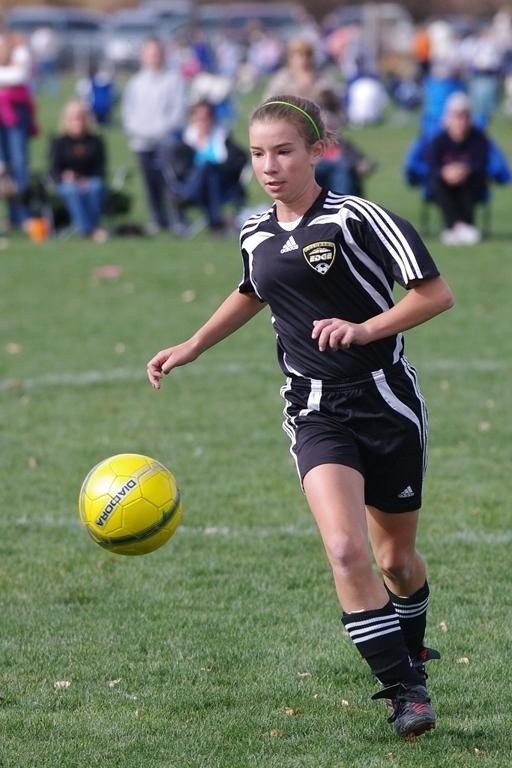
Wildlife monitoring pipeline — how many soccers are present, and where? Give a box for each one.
[79,454,182,555]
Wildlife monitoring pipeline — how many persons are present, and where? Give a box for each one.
[262,8,512,247]
[1,20,286,244]
[147,93,455,737]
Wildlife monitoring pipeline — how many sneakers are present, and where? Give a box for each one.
[371,649,441,740]
